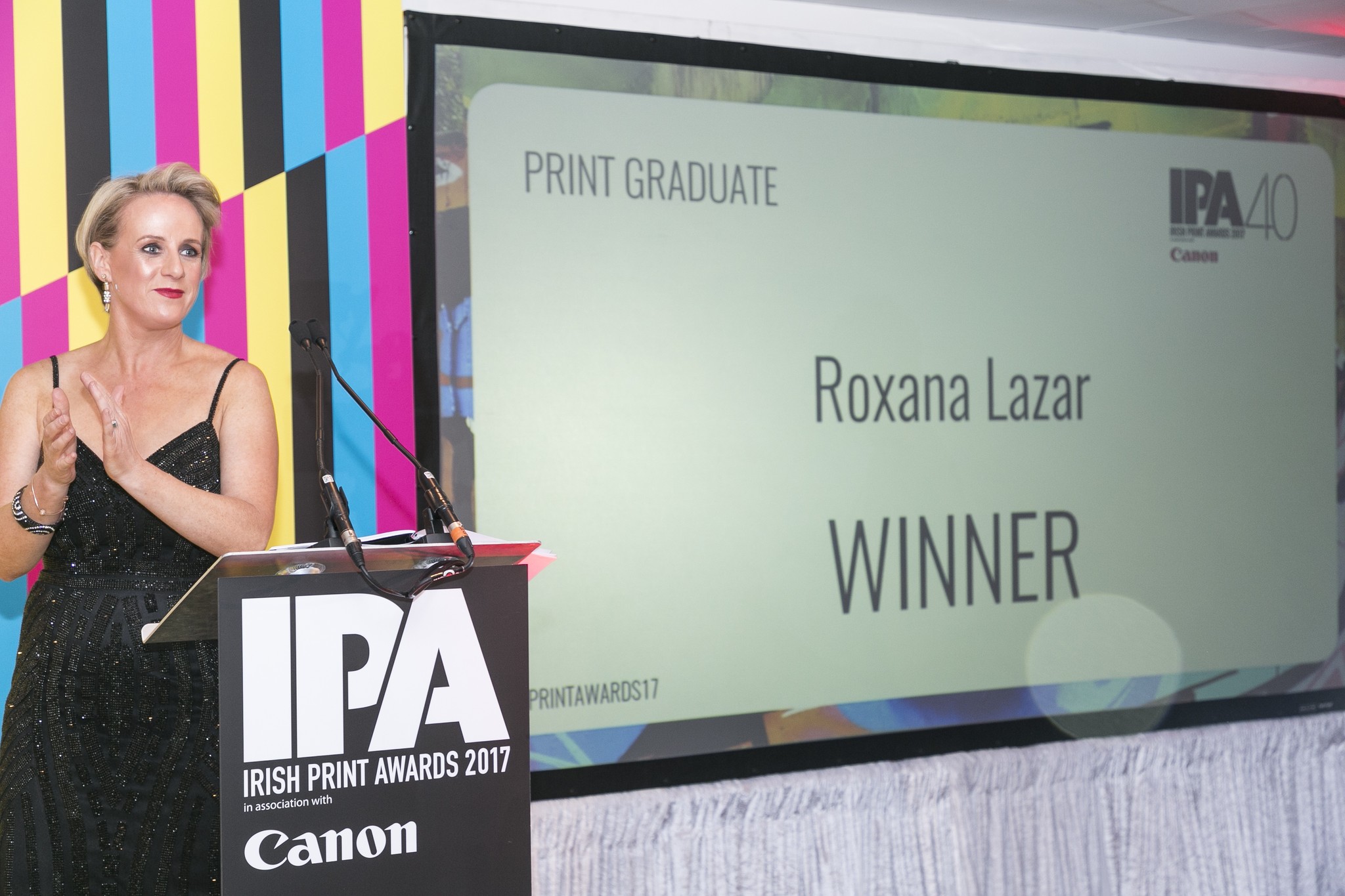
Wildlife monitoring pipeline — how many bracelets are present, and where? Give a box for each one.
[12,485,65,535]
[32,476,69,516]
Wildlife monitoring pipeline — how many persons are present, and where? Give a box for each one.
[0,162,280,896]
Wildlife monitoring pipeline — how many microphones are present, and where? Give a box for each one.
[288,318,363,567]
[306,318,474,558]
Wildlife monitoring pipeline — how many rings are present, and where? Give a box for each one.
[113,419,118,427]
